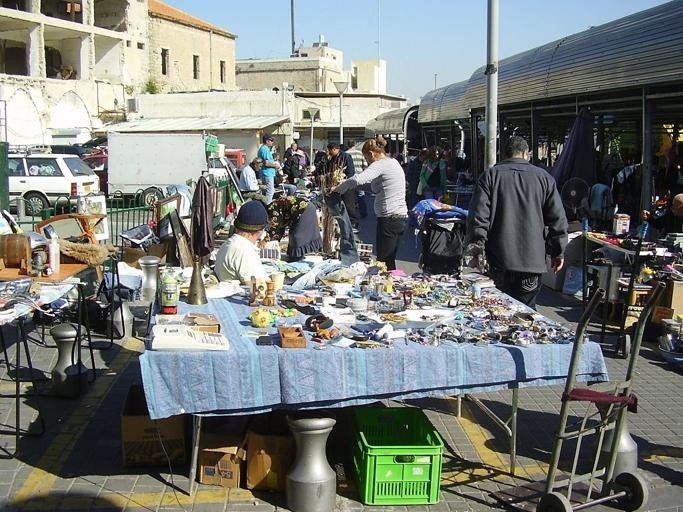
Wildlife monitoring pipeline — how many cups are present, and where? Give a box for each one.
[270,271,285,290]
[48,238,62,273]
[351,298,368,311]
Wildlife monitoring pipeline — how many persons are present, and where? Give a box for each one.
[468,135,568,309]
[214,199,272,291]
[611,155,683,236]
[239,134,467,229]
[256,197,323,260]
[331,137,407,270]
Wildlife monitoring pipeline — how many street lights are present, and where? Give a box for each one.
[333,76,348,144]
[307,105,318,168]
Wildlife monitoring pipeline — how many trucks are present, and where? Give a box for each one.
[107,134,229,209]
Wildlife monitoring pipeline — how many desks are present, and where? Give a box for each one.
[138,277,608,494]
[587,232,672,331]
[0,248,125,459]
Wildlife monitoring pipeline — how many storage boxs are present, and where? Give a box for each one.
[545,226,582,260]
[120,383,185,466]
[235,428,295,491]
[198,432,248,488]
[542,255,565,290]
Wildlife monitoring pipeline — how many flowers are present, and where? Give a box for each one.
[268,193,308,230]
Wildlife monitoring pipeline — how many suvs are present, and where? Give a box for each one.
[8,142,107,156]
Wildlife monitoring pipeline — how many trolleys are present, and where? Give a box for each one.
[536,283,666,512]
[579,217,647,358]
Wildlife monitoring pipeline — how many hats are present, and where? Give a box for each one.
[233,200,269,232]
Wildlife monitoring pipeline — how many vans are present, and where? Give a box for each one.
[7,154,100,216]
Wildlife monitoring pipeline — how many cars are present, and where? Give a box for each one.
[83,156,108,193]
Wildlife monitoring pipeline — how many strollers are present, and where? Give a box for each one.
[417,222,463,272]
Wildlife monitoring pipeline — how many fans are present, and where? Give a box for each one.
[561,177,590,221]
